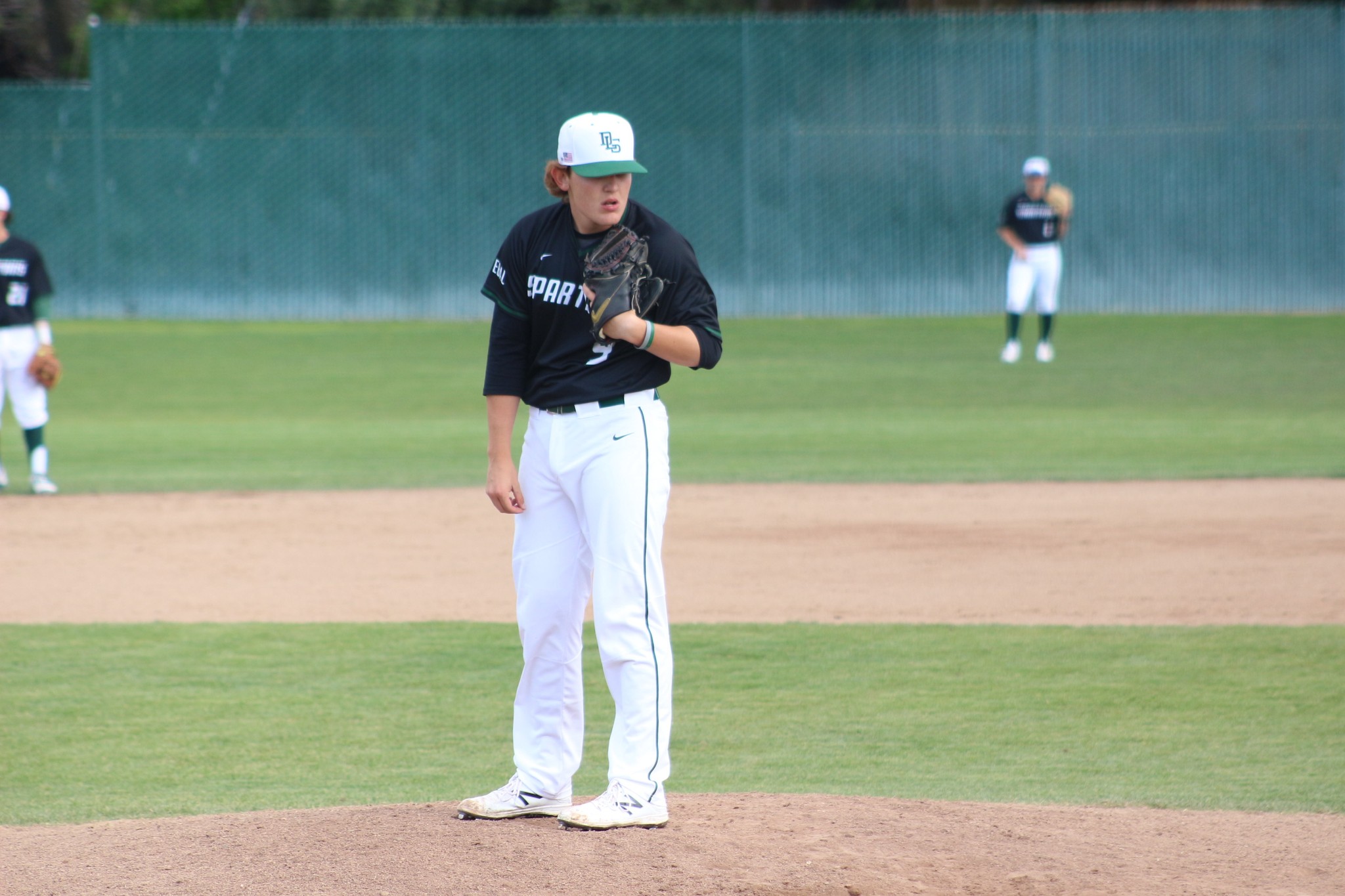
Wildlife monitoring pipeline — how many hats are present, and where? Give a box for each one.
[1022,157,1050,179]
[0,185,11,212]
[558,112,648,179]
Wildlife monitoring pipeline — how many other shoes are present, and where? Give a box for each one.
[1037,340,1054,362]
[1001,337,1020,364]
[0,466,7,488]
[31,474,58,493]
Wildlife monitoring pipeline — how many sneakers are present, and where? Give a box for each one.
[456,774,571,819]
[557,780,668,830]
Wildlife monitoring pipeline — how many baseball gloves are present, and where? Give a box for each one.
[29,348,58,388]
[583,226,663,344]
[1048,182,1072,213]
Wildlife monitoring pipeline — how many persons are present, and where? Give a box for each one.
[453,110,722,833]
[0,186,63,496]
[996,155,1074,364]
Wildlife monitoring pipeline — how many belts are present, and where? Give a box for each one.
[540,387,660,414]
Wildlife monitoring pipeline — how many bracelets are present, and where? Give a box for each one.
[633,320,655,350]
